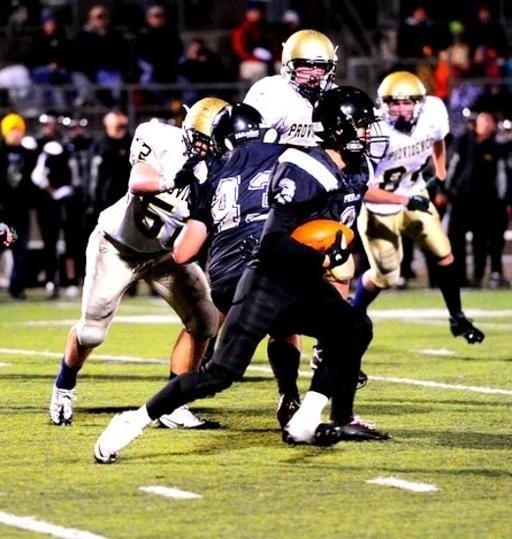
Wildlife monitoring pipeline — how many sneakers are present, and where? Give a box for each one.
[448,310,485,344]
[276,346,392,447]
[162,406,208,431]
[92,409,135,464]
[47,379,77,424]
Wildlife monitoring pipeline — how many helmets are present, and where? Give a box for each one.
[376,70,428,134]
[210,100,272,152]
[281,28,338,97]
[180,96,229,158]
[312,85,390,159]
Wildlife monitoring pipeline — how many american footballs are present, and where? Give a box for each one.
[291,219,355,253]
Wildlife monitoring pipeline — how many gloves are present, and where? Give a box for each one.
[175,154,212,202]
[326,232,351,270]
[406,195,430,216]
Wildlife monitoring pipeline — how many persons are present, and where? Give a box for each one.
[0,0,512,463]
